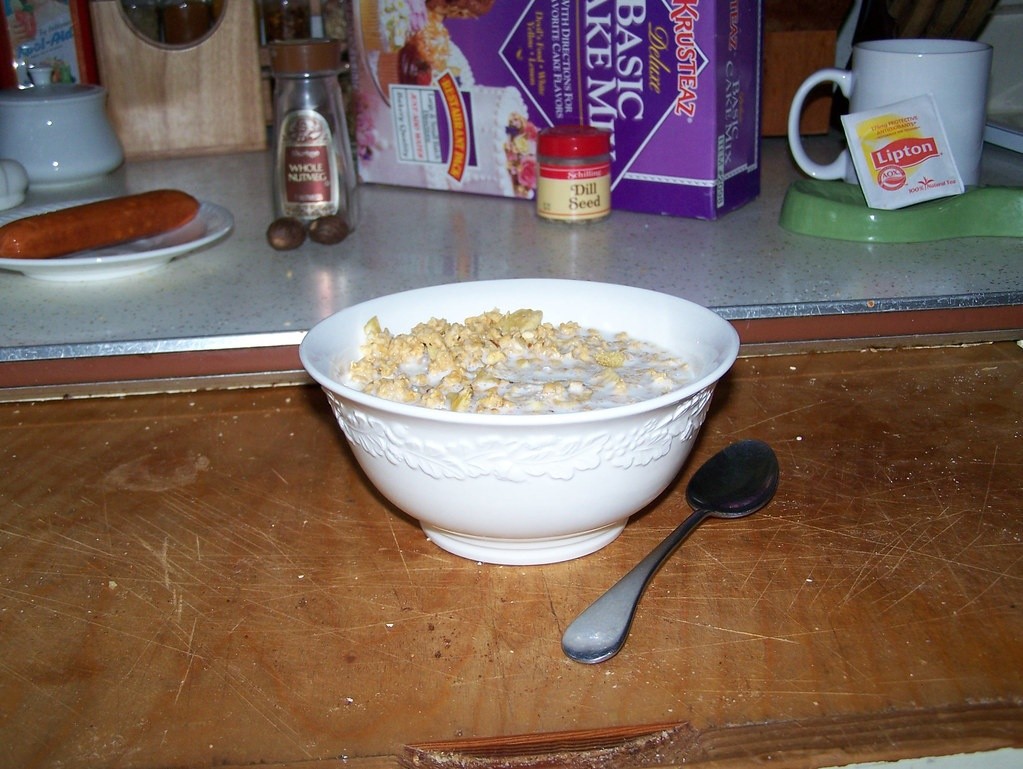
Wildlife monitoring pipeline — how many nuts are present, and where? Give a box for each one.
[267,216,350,250]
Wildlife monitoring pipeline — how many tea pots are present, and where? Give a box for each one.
[0,63,125,209]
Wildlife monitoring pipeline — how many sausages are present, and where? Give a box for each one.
[0,189,201,258]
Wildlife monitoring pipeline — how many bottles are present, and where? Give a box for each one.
[270,39,362,239]
[540,126,614,226]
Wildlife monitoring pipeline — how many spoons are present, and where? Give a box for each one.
[562,439,779,664]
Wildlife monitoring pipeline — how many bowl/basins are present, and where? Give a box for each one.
[299,279,741,565]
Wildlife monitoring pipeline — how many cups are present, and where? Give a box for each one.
[788,39,993,201]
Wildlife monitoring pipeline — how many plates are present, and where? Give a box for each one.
[0,201,233,281]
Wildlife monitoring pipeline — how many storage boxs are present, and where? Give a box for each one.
[344,0,767,223]
